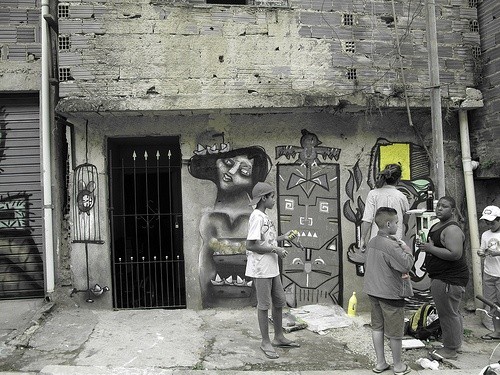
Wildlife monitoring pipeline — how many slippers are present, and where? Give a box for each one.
[373,362,393,373]
[426,347,458,361]
[272,339,301,348]
[395,364,412,375]
[260,345,280,359]
[481,332,500,340]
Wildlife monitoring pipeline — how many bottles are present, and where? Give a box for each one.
[348,292,358,316]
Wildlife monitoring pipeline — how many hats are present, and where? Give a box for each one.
[248,182,275,206]
[479,205,500,221]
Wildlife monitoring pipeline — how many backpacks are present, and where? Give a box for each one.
[404,302,442,342]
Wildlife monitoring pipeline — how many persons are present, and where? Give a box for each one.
[477,205,500,342]
[360,163,409,254]
[362,207,414,375]
[245,181,301,358]
[417,196,469,362]
